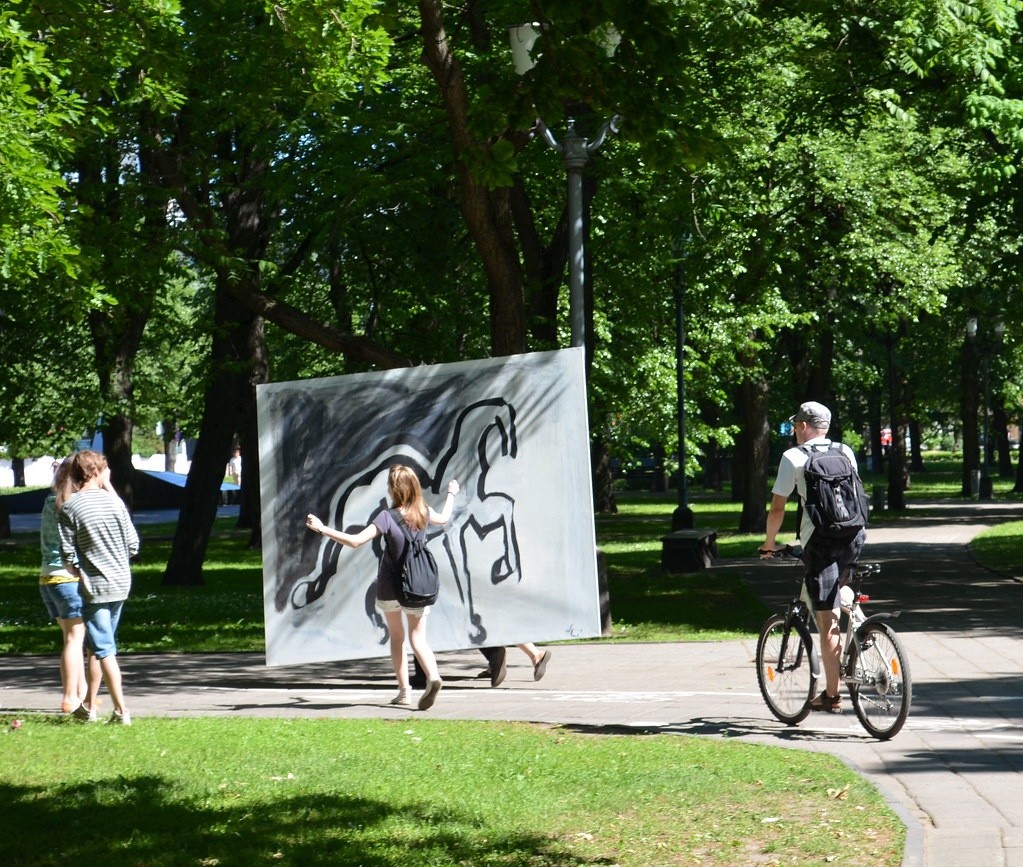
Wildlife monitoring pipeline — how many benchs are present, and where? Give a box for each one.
[660,528,719,574]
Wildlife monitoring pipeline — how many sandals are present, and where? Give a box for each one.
[803,689,843,714]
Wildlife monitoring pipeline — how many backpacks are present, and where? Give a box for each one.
[797,442,869,537]
[385,505,439,607]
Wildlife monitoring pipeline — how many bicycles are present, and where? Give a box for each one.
[750,546,914,739]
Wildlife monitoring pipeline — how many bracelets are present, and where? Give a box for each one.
[447,492,455,497]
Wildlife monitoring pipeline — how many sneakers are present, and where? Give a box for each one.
[71,703,97,722]
[103,711,130,726]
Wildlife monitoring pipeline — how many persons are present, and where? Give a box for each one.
[40,453,89,714]
[307,465,461,709]
[758,402,866,715]
[408,642,552,686]
[59,451,140,726]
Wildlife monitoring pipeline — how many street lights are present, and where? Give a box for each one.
[966,311,995,500]
[510,17,626,352]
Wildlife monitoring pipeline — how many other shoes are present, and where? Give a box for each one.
[418,674,442,710]
[391,686,412,705]
[62,695,103,712]
[534,651,551,681]
[491,648,506,687]
[409,675,426,689]
[478,670,491,677]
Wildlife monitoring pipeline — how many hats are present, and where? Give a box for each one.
[789,401,831,429]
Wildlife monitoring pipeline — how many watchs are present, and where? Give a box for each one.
[318,526,325,535]
